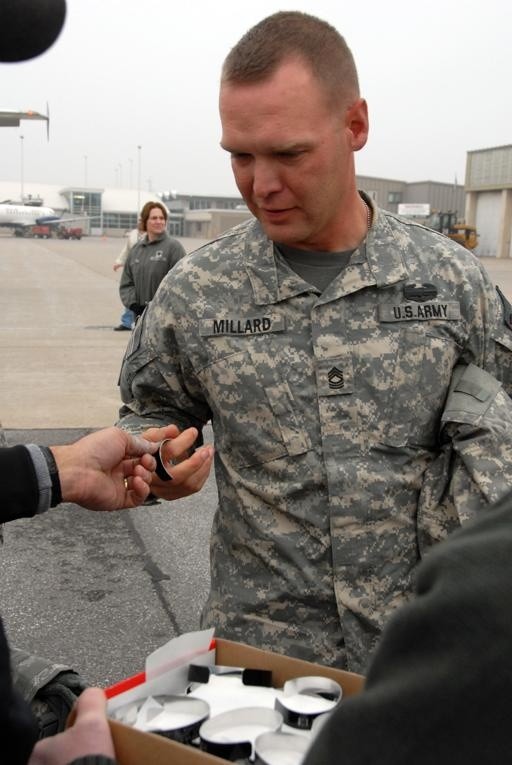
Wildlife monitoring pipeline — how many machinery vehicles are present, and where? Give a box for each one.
[423,209,476,251]
[31,224,82,241]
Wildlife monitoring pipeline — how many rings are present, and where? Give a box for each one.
[123,477,130,491]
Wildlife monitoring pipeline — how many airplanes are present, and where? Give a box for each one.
[1,205,60,238]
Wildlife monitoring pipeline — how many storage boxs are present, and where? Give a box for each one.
[65,637,368,764]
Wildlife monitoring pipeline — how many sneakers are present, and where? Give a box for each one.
[115,325,132,331]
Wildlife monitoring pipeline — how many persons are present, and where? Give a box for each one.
[111,213,149,332]
[0,423,163,764]
[117,199,186,324]
[25,488,510,764]
[116,7,512,680]
